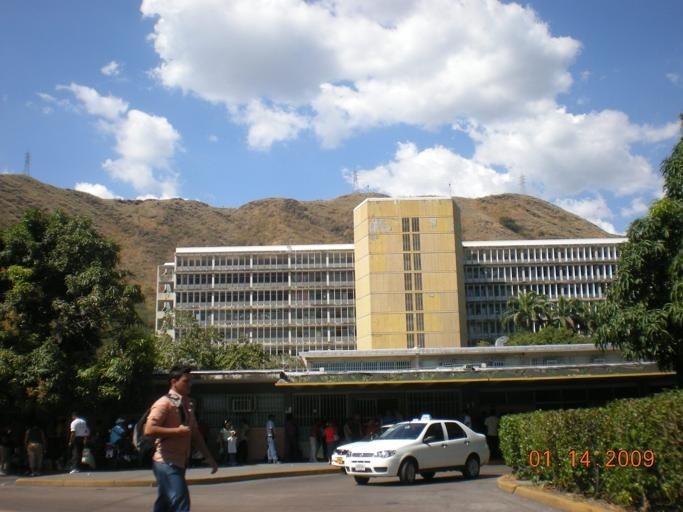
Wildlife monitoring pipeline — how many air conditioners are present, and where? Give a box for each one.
[232,398,252,413]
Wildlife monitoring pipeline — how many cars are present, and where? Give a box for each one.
[329,414,490,485]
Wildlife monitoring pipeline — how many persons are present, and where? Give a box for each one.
[309,414,378,463]
[265,415,281,465]
[3,411,154,476]
[483,408,502,460]
[461,408,473,430]
[192,413,250,467]
[141,363,218,512]
[284,414,302,461]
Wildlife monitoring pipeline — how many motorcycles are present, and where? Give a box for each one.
[104,424,137,466]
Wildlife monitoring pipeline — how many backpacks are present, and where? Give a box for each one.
[132,396,185,455]
